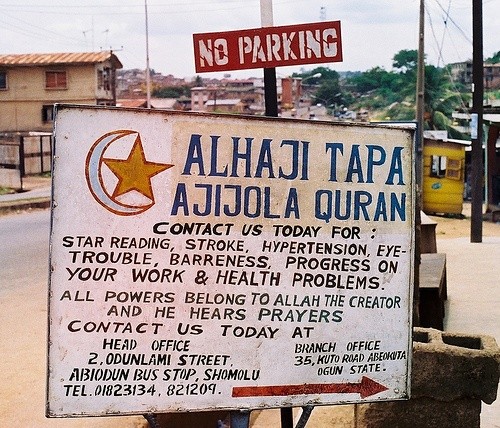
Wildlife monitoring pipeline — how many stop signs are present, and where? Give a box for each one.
[192,21,344,73]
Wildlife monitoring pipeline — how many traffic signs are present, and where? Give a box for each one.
[45,104,418,418]
